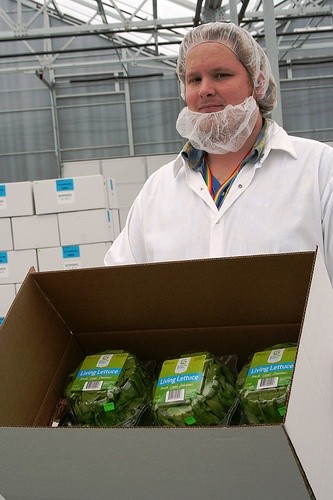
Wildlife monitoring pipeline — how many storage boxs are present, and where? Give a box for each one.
[0,173,120,329]
[0,243,333,500]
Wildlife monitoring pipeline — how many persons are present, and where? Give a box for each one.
[98,21,333,293]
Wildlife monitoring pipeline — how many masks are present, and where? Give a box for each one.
[175,97,260,154]
